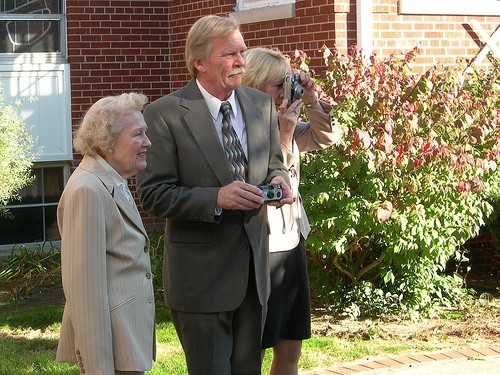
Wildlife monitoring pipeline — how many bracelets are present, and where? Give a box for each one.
[303,94,318,108]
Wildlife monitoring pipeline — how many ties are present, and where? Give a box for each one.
[220,101,248,184]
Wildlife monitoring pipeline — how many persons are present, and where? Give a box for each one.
[137,14,294,375]
[240,48,342,375]
[56,92,157,375]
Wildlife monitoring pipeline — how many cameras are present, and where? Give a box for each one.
[256,183,283,202]
[283,73,305,108]
